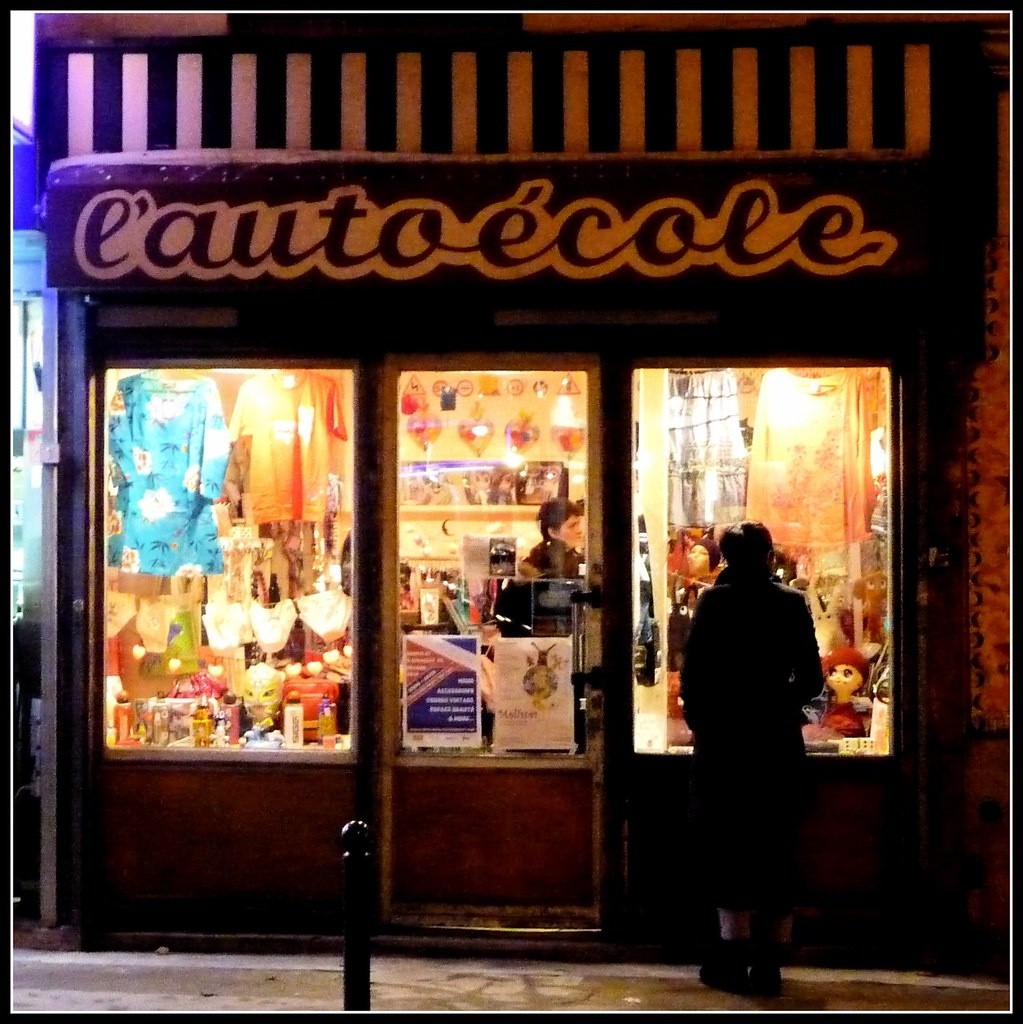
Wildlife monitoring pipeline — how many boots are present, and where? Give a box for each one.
[700,938,783,997]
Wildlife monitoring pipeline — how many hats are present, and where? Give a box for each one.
[690,539,721,573]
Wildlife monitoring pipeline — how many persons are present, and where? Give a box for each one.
[635,509,660,688]
[522,498,583,586]
[681,524,828,995]
[687,537,722,578]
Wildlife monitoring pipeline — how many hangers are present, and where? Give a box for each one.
[141,368,199,393]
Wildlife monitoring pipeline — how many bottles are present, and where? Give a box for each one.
[113,691,132,742]
[220,693,240,743]
[283,699,305,750]
[193,706,212,749]
[132,698,151,740]
[152,691,170,747]
